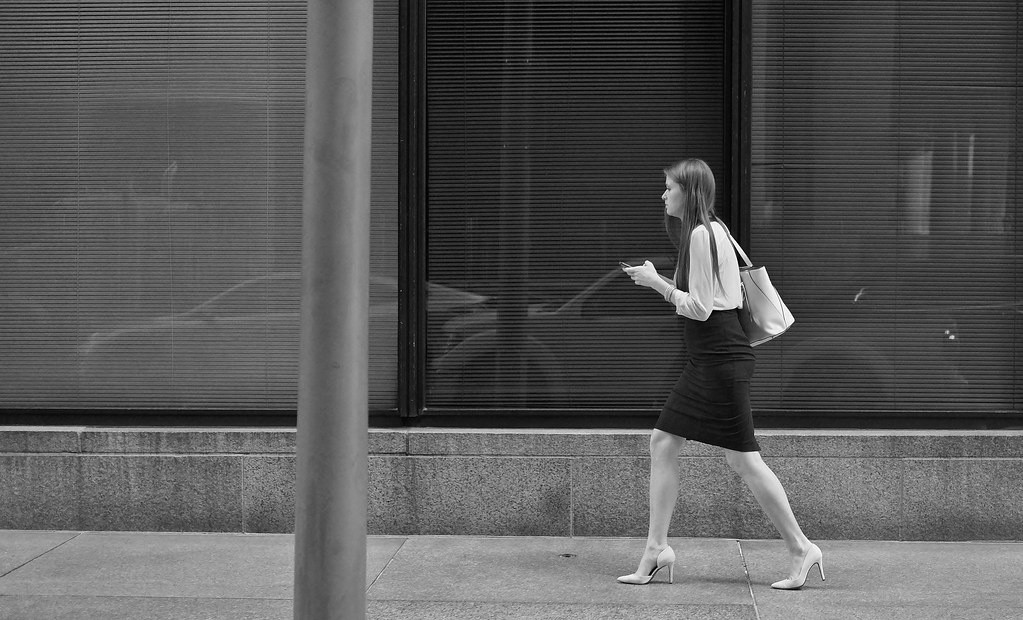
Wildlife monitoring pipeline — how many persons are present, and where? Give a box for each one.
[617,158,826,590]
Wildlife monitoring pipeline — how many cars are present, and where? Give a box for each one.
[72,267,497,416]
[428,254,813,410]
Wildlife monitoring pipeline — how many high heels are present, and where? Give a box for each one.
[618,547,676,585]
[771,544,825,589]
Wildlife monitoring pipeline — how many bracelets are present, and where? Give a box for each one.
[664,284,676,302]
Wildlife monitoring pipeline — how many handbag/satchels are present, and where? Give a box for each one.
[710,216,795,348]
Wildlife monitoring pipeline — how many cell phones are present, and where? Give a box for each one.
[619,262,631,268]
